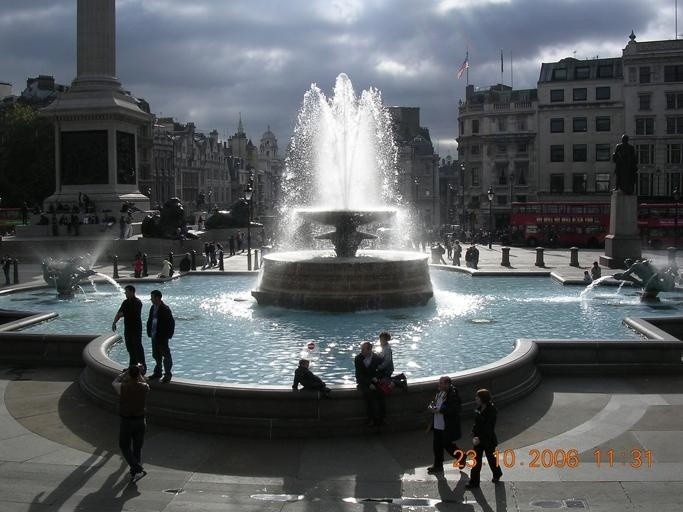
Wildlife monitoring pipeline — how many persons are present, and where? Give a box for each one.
[620,258,678,303]
[111,363,147,484]
[425,375,466,471]
[353,341,386,427]
[159,229,245,277]
[470,231,491,245]
[110,285,148,374]
[198,209,211,230]
[612,134,638,192]
[7,194,142,239]
[437,235,461,266]
[462,230,467,244]
[584,262,600,285]
[465,388,502,488]
[146,289,175,383]
[132,251,144,277]
[375,332,408,391]
[292,360,330,395]
[256,229,266,247]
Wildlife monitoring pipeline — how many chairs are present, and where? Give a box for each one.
[127,471,147,488]
[459,452,467,469]
[427,467,443,472]
[465,482,480,488]
[492,471,503,483]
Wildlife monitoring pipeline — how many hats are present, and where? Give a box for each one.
[426,221,475,244]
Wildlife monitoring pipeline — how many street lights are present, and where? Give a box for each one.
[377,378,395,395]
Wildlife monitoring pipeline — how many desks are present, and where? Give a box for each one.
[459,162,466,230]
[413,175,420,208]
[486,184,495,250]
[671,184,681,246]
[242,182,252,271]
[146,185,151,198]
[249,168,255,222]
[207,187,213,210]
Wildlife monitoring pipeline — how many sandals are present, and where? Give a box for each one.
[0,207,42,222]
[638,201,682,246]
[508,198,611,246]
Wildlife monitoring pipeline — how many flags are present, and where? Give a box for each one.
[454,54,469,80]
[500,50,504,73]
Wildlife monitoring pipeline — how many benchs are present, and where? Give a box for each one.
[454,54,469,80]
[500,50,504,73]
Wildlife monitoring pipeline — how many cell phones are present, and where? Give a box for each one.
[136,363,142,369]
